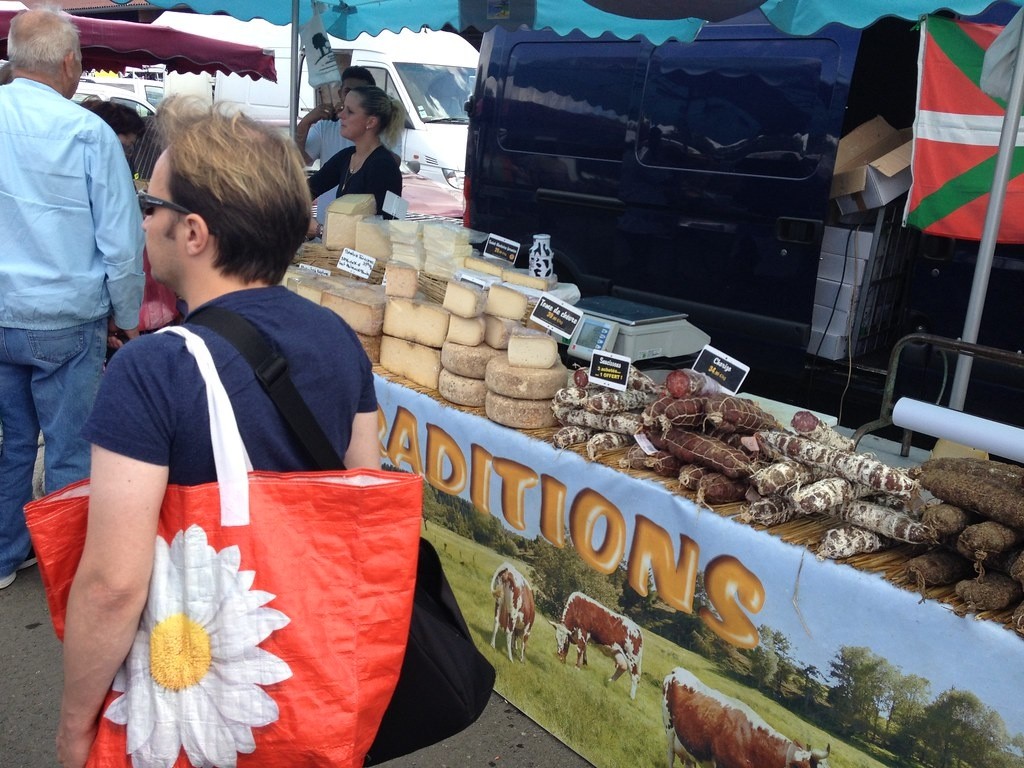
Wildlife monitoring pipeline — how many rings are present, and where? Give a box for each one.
[324,109,330,113]
[340,106,344,109]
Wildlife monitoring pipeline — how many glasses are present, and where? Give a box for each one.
[139,190,220,236]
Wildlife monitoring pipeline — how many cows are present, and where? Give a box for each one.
[661,665,831,768]
[546,590,645,702]
[488,561,537,665]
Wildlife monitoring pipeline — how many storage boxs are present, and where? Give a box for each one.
[807,225,916,359]
[831,115,913,215]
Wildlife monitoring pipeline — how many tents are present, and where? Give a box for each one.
[0,10,280,105]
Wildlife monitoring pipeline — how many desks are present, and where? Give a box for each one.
[369,360,1024,768]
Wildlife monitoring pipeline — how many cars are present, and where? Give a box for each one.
[71,60,166,126]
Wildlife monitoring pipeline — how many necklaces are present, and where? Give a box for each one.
[341,143,378,190]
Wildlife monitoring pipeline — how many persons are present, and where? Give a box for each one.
[55,92,385,768]
[307,86,403,238]
[0,6,145,589]
[294,66,403,240]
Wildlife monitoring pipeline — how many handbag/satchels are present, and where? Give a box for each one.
[25,324,422,768]
[361,538,496,768]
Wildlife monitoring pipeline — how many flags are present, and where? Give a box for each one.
[903,17,1023,244]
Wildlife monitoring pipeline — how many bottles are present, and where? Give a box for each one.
[529,234,552,278]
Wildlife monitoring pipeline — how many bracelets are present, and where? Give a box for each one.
[316,224,323,238]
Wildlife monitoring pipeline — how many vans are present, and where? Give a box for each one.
[461,24,1024,466]
[163,11,481,201]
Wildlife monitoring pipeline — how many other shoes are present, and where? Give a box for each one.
[0,544,38,590]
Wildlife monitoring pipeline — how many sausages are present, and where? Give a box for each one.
[548,368,1024,637]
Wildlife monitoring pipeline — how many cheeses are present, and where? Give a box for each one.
[285,192,567,429]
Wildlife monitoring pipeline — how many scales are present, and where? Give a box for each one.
[550,294,711,370]
